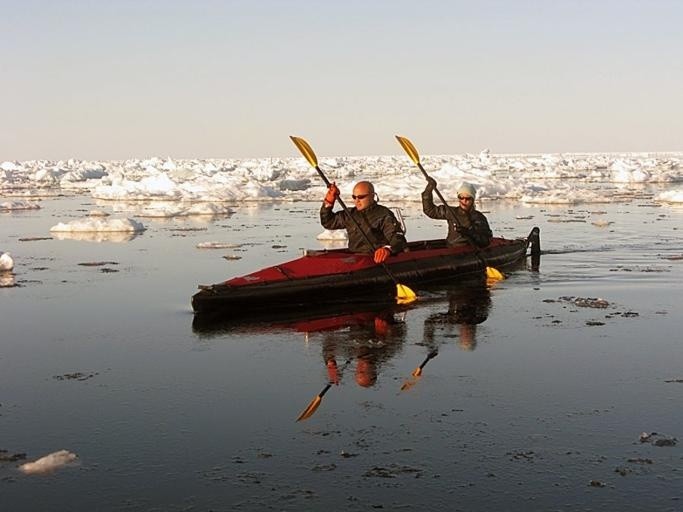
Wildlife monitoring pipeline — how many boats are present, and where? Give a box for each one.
[192,237,529,316]
[193,278,486,342]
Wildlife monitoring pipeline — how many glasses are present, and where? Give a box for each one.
[458,194,472,200]
[352,193,379,202]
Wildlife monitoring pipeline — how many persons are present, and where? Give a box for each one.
[421,175,493,248]
[322,314,408,387]
[421,289,491,356]
[319,180,407,264]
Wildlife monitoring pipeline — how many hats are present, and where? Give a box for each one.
[457,182,476,199]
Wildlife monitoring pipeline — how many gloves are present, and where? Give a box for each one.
[374,247,392,264]
[325,182,340,204]
[454,222,469,239]
[426,177,437,192]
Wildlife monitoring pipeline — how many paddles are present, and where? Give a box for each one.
[398,137,505,284]
[290,136,418,304]
[294,349,440,423]
